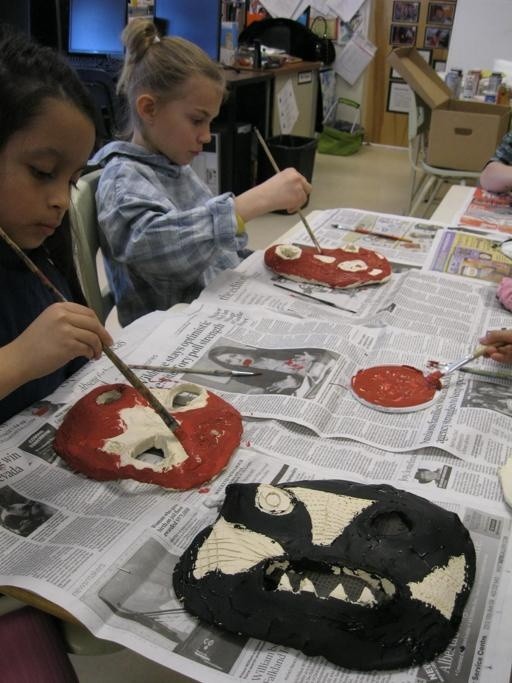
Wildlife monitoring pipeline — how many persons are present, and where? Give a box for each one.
[469,384,512,414]
[208,345,320,395]
[394,3,452,48]
[478,88,512,194]
[86,15,314,330]
[414,468,443,486]
[1,34,115,681]
[479,328,512,365]
[120,577,194,638]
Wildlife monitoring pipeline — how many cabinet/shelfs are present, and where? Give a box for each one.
[223,62,323,193]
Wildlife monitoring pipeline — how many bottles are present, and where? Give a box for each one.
[444,69,502,104]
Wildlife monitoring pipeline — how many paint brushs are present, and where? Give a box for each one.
[431,341,507,386]
[273,283,356,313]
[128,364,262,376]
[0,227,196,461]
[331,224,413,243]
[253,126,322,254]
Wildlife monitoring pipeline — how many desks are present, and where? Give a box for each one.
[1,207,511,682]
[429,185,477,223]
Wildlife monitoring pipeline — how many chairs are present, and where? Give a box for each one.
[75,68,127,147]
[69,168,115,326]
[408,88,481,217]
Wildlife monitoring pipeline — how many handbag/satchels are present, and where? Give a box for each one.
[317,98,364,156]
[311,16,335,65]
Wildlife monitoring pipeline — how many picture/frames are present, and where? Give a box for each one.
[390,47,432,79]
[426,2,456,26]
[423,26,452,49]
[389,23,418,48]
[392,0,421,23]
[387,81,409,114]
[432,60,447,72]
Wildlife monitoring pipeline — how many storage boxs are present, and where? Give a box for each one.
[387,47,512,172]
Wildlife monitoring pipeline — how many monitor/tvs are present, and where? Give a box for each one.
[68,0,127,56]
[154,0,222,63]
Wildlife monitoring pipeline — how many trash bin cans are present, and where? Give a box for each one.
[265,134,318,216]
